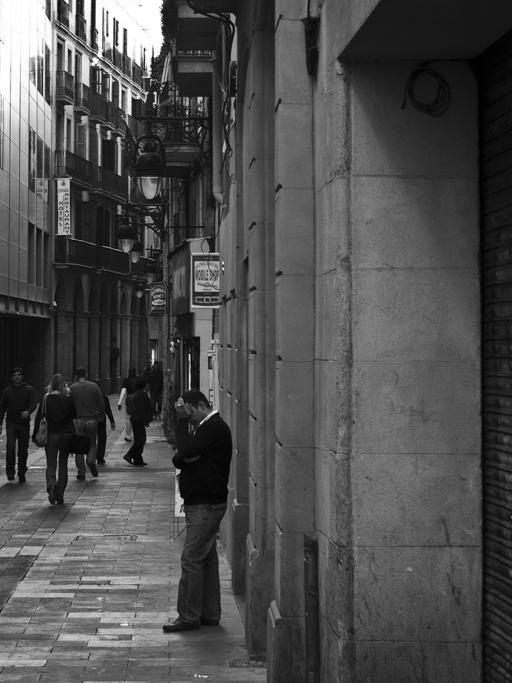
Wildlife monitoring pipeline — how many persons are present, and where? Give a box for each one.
[162,390,232,632]
[0,358,162,505]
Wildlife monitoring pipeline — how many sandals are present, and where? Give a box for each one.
[124,437,131,442]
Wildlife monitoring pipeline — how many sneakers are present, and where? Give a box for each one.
[122,454,147,467]
[76,474,85,480]
[6,473,26,483]
[87,462,98,477]
[161,616,218,632]
[48,486,64,505]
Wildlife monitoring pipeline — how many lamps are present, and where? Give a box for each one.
[117,116,213,299]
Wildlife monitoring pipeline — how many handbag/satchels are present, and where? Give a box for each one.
[34,397,48,448]
[68,434,91,454]
